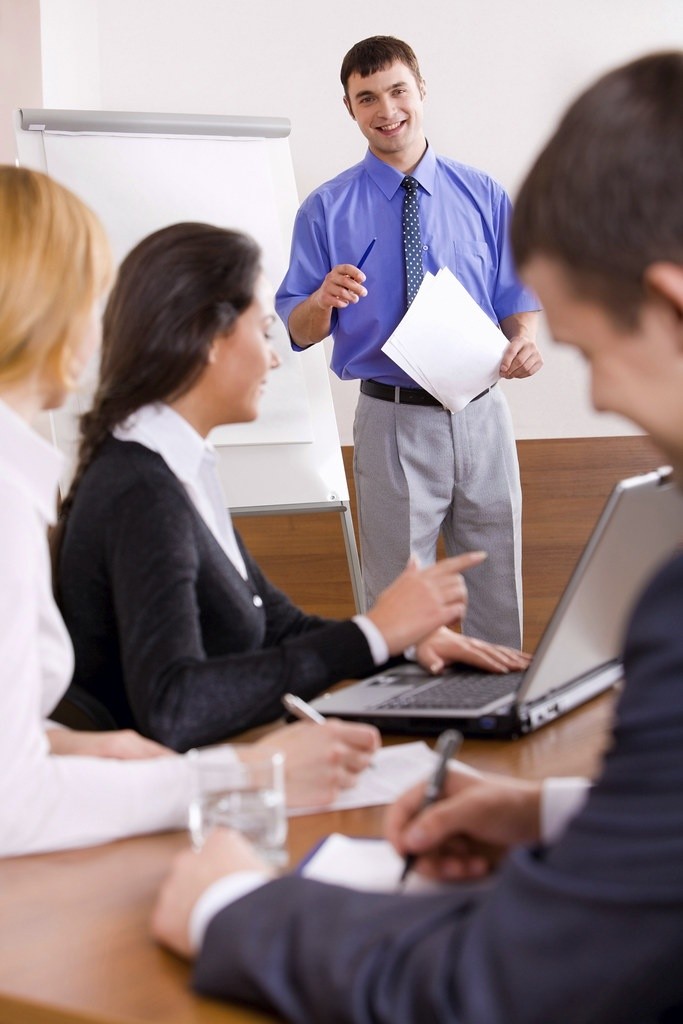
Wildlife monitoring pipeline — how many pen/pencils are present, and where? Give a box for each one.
[344,238,377,291]
[398,727,463,885]
[280,692,382,771]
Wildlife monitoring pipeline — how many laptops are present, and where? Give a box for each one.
[286,464,683,736]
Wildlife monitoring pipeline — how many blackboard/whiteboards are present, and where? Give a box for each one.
[12,103,353,516]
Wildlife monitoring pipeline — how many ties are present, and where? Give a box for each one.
[398,170,426,309]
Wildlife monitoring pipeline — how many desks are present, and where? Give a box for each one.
[1,677,625,1024]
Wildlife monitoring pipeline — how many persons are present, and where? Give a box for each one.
[149,53,683,1023]
[273,36,547,668]
[48,220,534,755]
[0,166,383,859]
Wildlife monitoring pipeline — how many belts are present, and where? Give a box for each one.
[356,376,501,406]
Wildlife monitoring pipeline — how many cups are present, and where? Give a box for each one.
[185,743,287,851]
